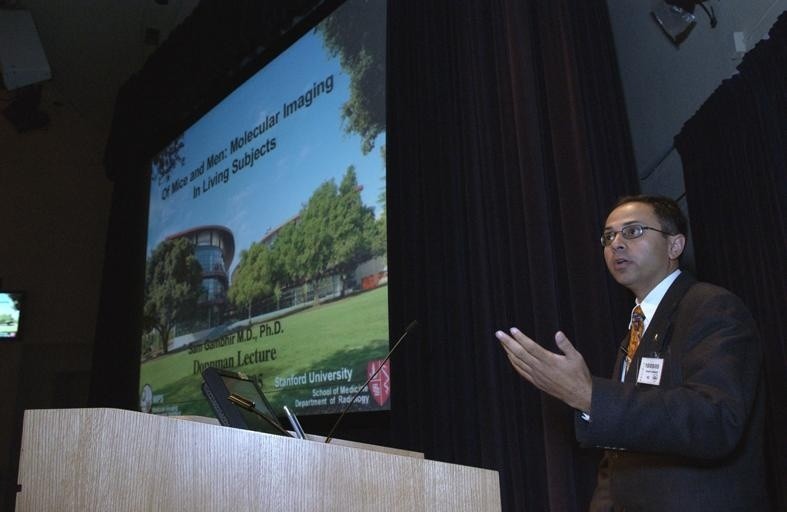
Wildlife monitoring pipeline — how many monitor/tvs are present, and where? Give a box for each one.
[201,367,287,436]
[0,288,27,343]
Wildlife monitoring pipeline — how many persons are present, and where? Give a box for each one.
[496,194,772,512]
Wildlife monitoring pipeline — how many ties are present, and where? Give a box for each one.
[625,306,645,375]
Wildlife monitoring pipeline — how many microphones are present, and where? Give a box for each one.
[324,320,418,443]
[226,393,296,437]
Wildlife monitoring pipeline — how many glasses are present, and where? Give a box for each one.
[600,224,673,247]
